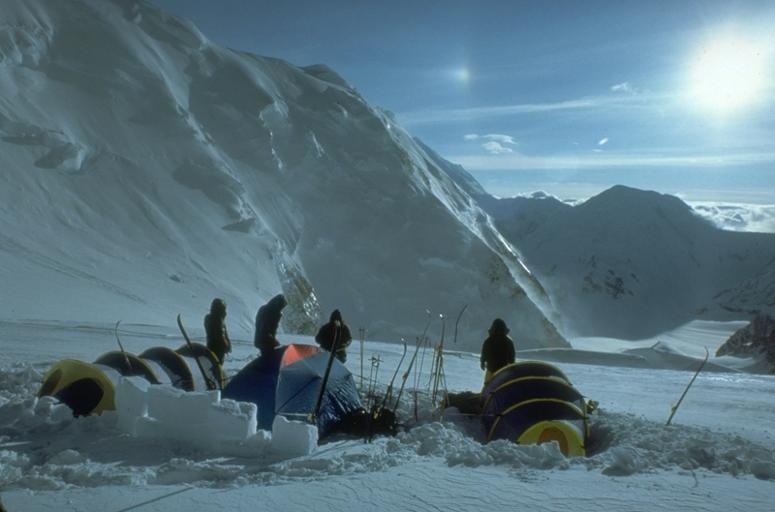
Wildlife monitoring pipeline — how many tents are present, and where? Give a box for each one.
[220,344,363,441]
[37,343,226,418]
[481,361,589,459]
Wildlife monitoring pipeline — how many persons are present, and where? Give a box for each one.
[255,294,288,355]
[202,298,232,364]
[316,309,353,364]
[481,319,517,381]
